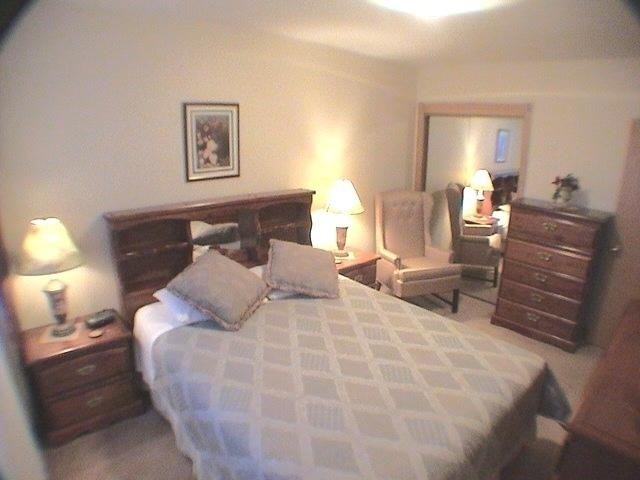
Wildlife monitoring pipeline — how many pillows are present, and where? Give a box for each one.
[153,239,340,331]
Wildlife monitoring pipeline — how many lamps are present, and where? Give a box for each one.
[326,179,365,257]
[12,217,82,337]
[470,170,494,218]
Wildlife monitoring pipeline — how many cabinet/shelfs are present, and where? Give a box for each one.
[464,218,500,236]
[491,196,615,353]
[555,299,640,480]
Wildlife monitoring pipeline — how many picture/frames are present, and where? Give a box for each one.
[495,129,510,164]
[184,103,240,182]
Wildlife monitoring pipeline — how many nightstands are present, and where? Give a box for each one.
[326,246,381,289]
[16,309,146,448]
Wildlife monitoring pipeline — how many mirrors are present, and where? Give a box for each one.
[414,103,532,305]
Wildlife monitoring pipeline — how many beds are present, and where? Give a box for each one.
[490,171,519,253]
[102,188,573,480]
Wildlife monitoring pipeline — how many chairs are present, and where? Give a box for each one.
[375,190,465,313]
[446,183,506,288]
